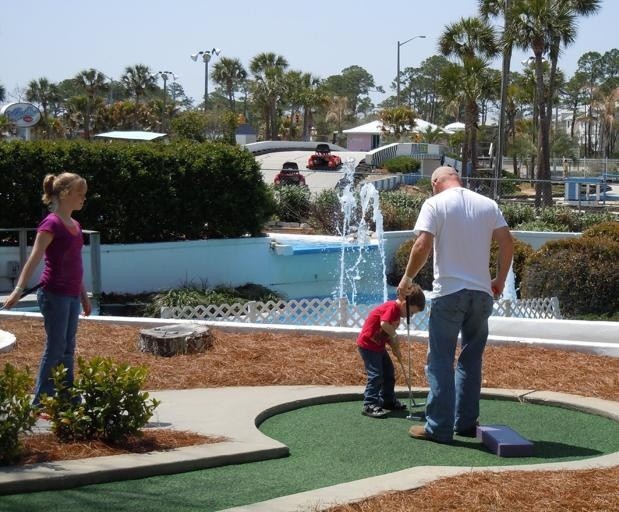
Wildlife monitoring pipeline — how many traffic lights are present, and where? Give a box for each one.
[295,113,300,124]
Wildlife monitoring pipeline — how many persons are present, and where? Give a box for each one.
[359,282,426,420]
[397,166,513,442]
[4,169,92,423]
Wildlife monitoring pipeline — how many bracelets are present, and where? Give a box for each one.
[13,286,25,293]
[404,272,413,281]
[392,332,397,338]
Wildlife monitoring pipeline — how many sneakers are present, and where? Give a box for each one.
[383,402,407,409]
[409,425,428,439]
[361,404,387,418]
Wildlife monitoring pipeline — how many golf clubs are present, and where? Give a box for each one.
[406,296,422,422]
[395,355,425,408]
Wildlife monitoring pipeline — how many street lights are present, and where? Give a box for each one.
[151,70,180,134]
[395,36,426,135]
[190,48,221,115]
[520,55,548,189]
[90,68,113,110]
